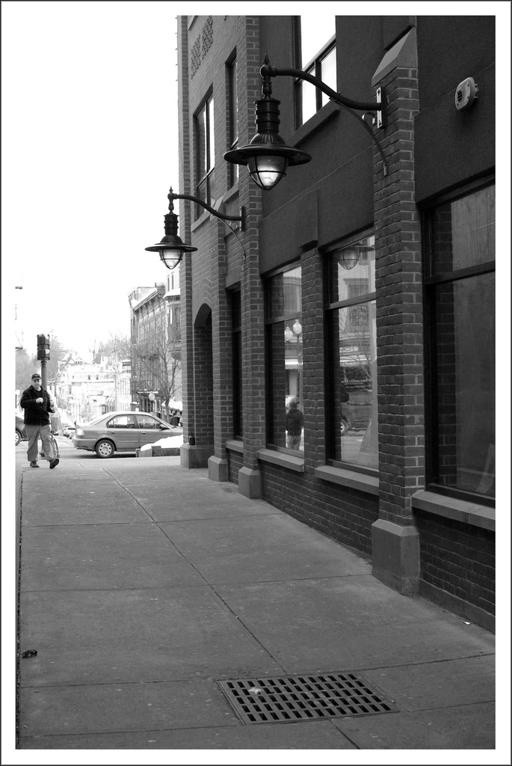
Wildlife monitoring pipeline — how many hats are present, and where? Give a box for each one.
[32,374,41,380]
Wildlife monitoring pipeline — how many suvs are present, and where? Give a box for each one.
[335,379,377,437]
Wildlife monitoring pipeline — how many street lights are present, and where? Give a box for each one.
[148,390,156,416]
[285,317,305,426]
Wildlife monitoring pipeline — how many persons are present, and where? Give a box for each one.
[20,373,60,469]
[284,398,303,451]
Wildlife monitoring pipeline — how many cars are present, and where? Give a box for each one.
[72,411,185,459]
[284,392,304,432]
[15,412,81,452]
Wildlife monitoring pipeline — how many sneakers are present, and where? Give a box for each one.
[50,459,59,469]
[31,461,39,467]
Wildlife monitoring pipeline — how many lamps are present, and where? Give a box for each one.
[336,243,361,271]
[223,53,389,191]
[145,186,247,267]
